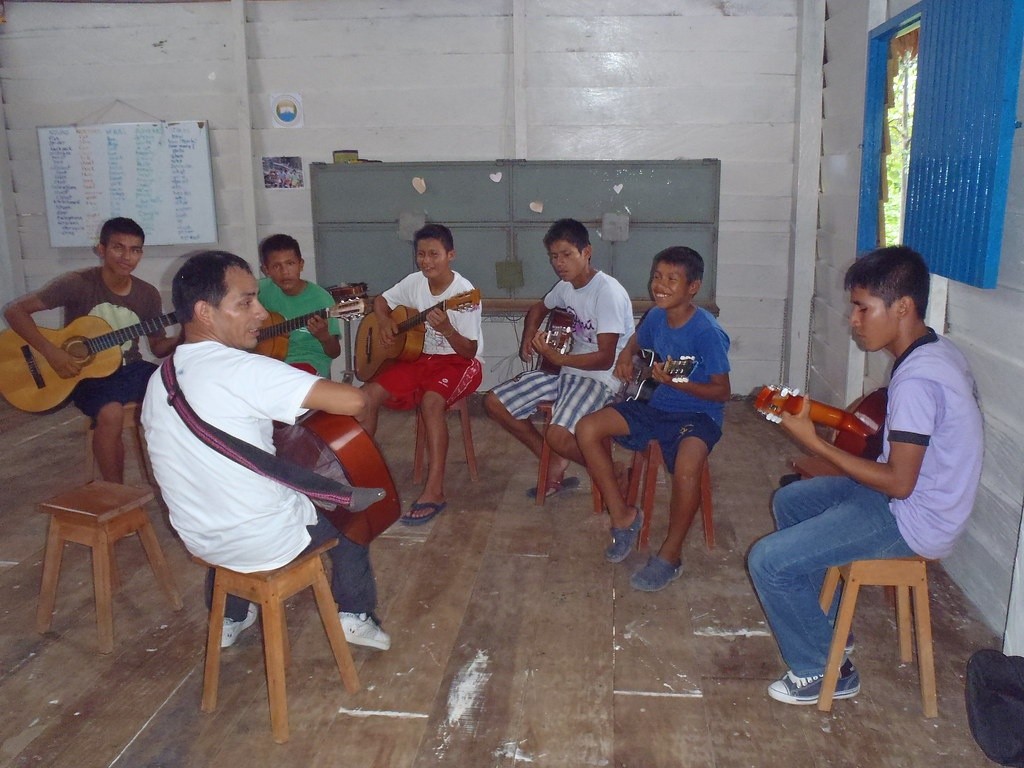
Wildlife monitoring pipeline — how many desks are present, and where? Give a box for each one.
[341,301,720,386]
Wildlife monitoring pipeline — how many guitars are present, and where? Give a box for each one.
[353,287,481,383]
[250,296,369,362]
[272,408,403,546]
[534,308,575,375]
[622,347,704,405]
[1,310,180,416]
[752,383,889,461]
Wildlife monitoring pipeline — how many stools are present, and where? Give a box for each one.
[36,482,183,654]
[535,403,714,549]
[413,396,478,484]
[818,556,939,718]
[85,401,150,484]
[192,539,360,743]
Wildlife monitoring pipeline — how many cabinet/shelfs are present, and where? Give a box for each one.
[310,158,720,301]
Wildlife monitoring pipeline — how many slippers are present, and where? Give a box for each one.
[527,476,579,498]
[630,554,684,591]
[399,500,448,525]
[605,507,642,562]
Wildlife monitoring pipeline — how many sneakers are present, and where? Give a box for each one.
[339,611,391,650]
[845,631,855,655]
[221,602,258,647]
[768,666,861,705]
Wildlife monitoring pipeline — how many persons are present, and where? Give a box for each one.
[256,234,342,378]
[279,168,299,188]
[747,245,985,705]
[140,250,391,651]
[484,217,636,500]
[355,224,485,526]
[3,217,185,485]
[575,246,732,591]
